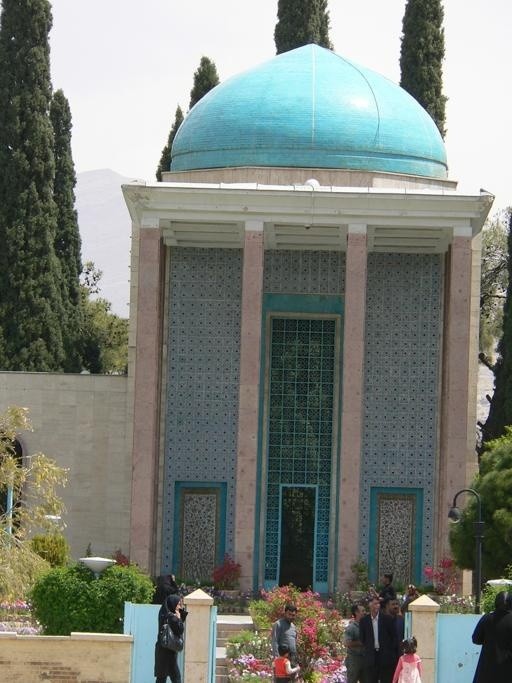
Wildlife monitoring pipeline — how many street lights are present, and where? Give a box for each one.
[446,485,486,613]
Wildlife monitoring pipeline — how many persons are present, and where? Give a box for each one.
[152,574,179,604]
[272,645,300,683]
[471,591,512,683]
[154,594,188,683]
[344,573,422,683]
[272,604,300,683]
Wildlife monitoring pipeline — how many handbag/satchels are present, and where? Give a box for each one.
[159,624,183,652]
